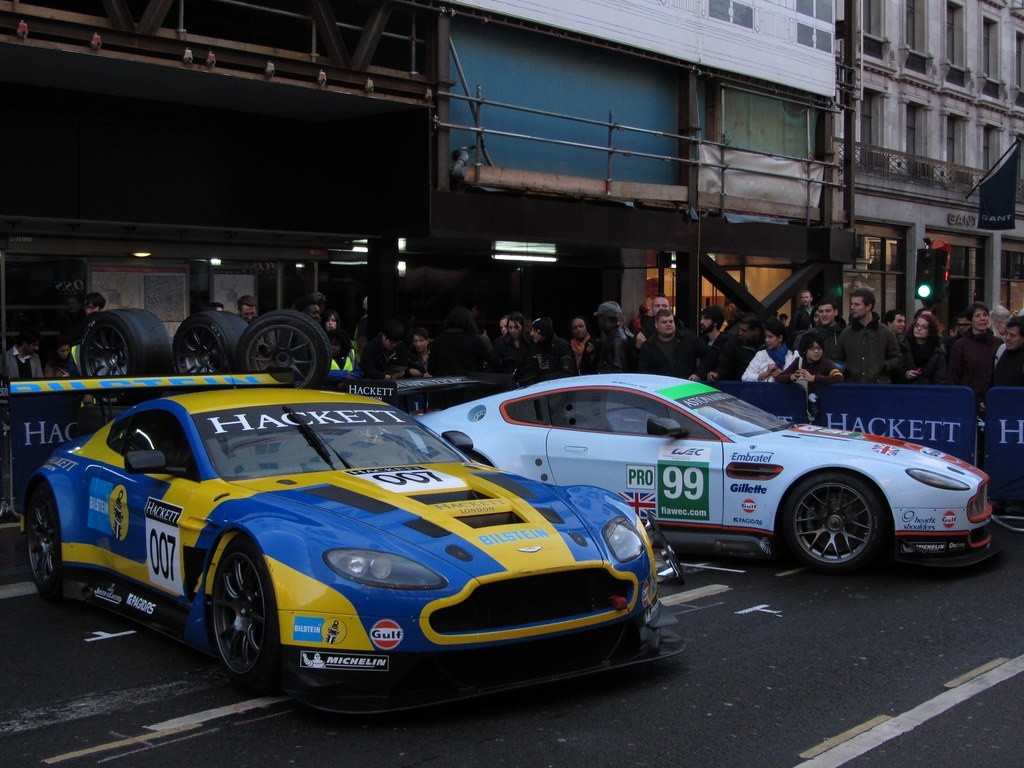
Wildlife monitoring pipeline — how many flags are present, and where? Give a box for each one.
[976,146,1021,230]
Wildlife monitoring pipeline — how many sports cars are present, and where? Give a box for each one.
[6,373,687,714]
[407,373,1001,576]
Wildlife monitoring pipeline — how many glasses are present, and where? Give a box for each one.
[915,323,929,329]
[808,345,822,350]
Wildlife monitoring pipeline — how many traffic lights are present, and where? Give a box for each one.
[936,267,949,288]
[913,247,935,300]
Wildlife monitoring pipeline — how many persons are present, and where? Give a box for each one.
[290,297,719,394]
[203,302,224,313]
[239,294,277,368]
[698,289,1024,422]
[83,292,106,315]
[0,328,43,378]
[56,294,86,342]
[44,336,80,377]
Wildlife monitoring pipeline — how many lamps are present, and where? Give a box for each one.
[492,241,559,262]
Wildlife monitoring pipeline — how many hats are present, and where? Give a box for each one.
[640,304,649,312]
[594,301,623,318]
[991,305,1010,323]
[533,317,553,338]
